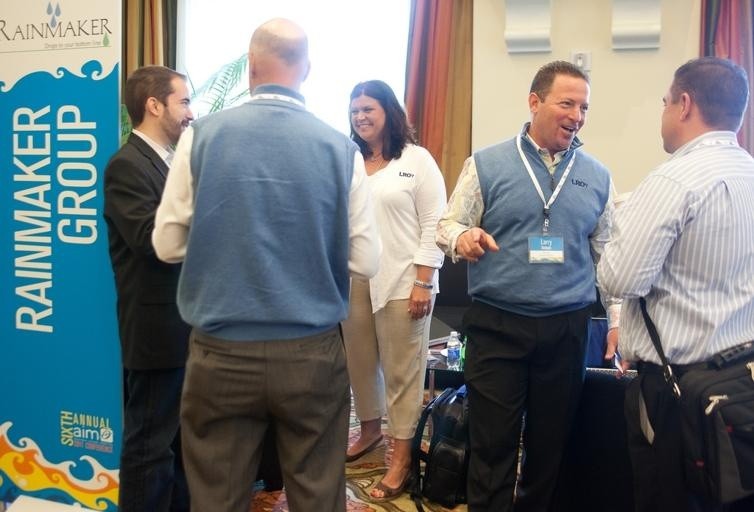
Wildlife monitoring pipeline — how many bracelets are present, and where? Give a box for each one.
[413,280,433,289]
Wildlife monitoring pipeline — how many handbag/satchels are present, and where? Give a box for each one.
[675,338,754,506]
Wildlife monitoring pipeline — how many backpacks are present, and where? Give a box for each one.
[410,384,471,512]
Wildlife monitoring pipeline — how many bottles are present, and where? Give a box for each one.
[446,331,461,369]
[460,337,467,365]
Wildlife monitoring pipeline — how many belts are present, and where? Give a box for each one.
[636,360,709,380]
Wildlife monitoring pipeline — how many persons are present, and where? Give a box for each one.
[104,63,195,510]
[594,53,753,512]
[151,17,380,512]
[433,59,631,512]
[343,76,448,501]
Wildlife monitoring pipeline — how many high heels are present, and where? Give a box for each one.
[345,434,386,468]
[370,470,414,503]
